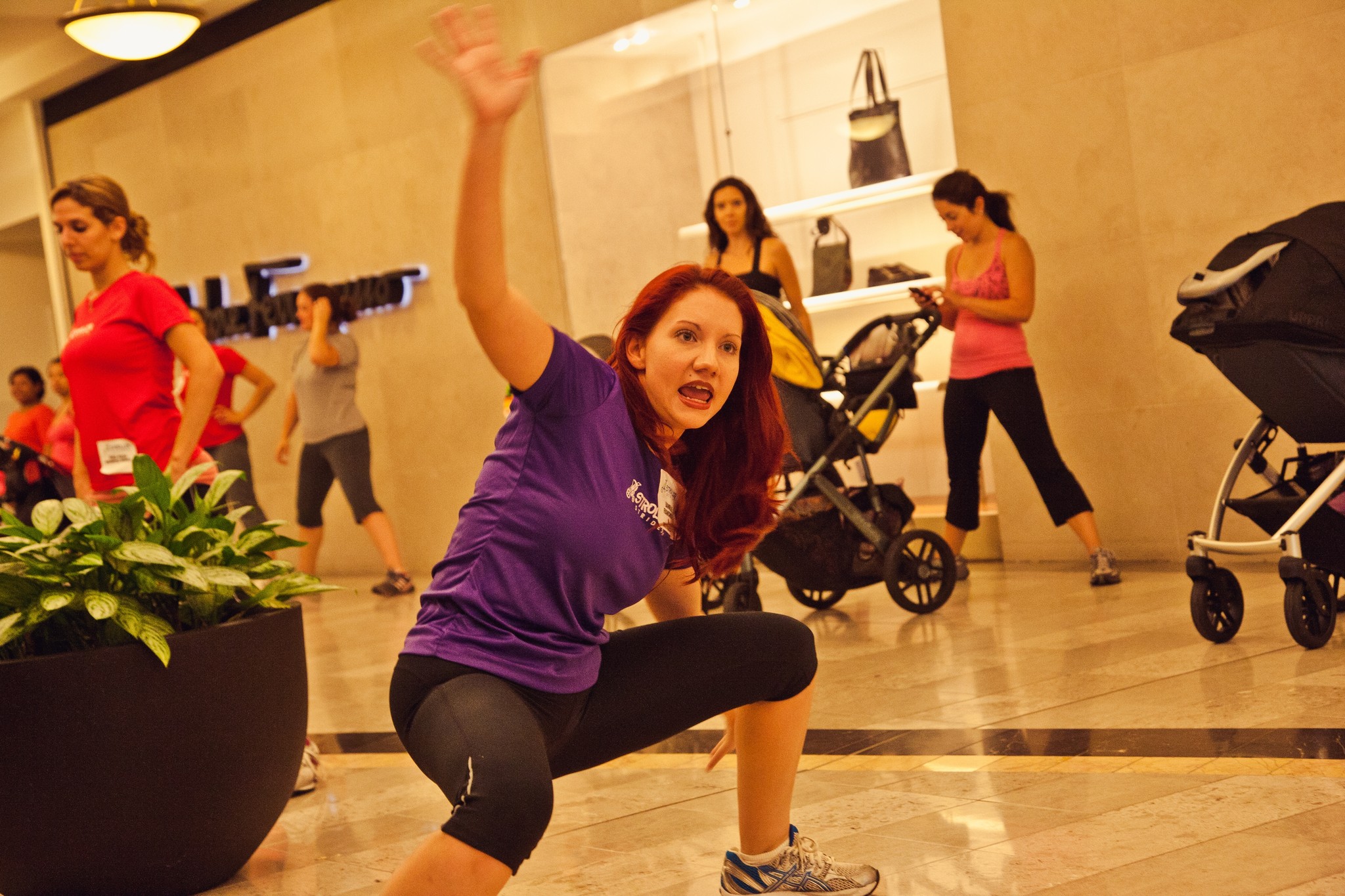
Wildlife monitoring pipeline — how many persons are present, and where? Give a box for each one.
[49,357,75,472]
[389,5,880,895]
[50,176,324,796]
[702,179,811,341]
[179,306,276,560]
[273,283,416,596]
[909,170,1119,590]
[5,367,55,453]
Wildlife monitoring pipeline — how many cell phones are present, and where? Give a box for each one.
[909,288,939,308]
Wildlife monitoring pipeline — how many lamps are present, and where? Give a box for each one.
[57,0,206,60]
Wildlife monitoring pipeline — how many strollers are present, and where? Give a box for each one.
[0,435,71,532]
[1167,199,1345,649]
[699,307,960,618]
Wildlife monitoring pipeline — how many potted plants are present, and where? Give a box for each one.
[0,455,347,896]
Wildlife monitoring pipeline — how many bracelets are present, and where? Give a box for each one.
[166,454,188,467]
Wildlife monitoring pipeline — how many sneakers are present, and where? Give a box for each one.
[371,566,416,596]
[719,822,881,896]
[902,554,971,583]
[1089,542,1119,586]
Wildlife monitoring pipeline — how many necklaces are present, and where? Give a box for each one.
[721,241,753,260]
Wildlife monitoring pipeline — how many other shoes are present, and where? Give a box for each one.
[293,736,319,793]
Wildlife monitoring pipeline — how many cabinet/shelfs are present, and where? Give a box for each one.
[680,168,948,400]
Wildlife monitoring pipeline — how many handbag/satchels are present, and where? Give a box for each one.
[846,48,912,190]
[866,264,931,288]
[808,216,853,298]
